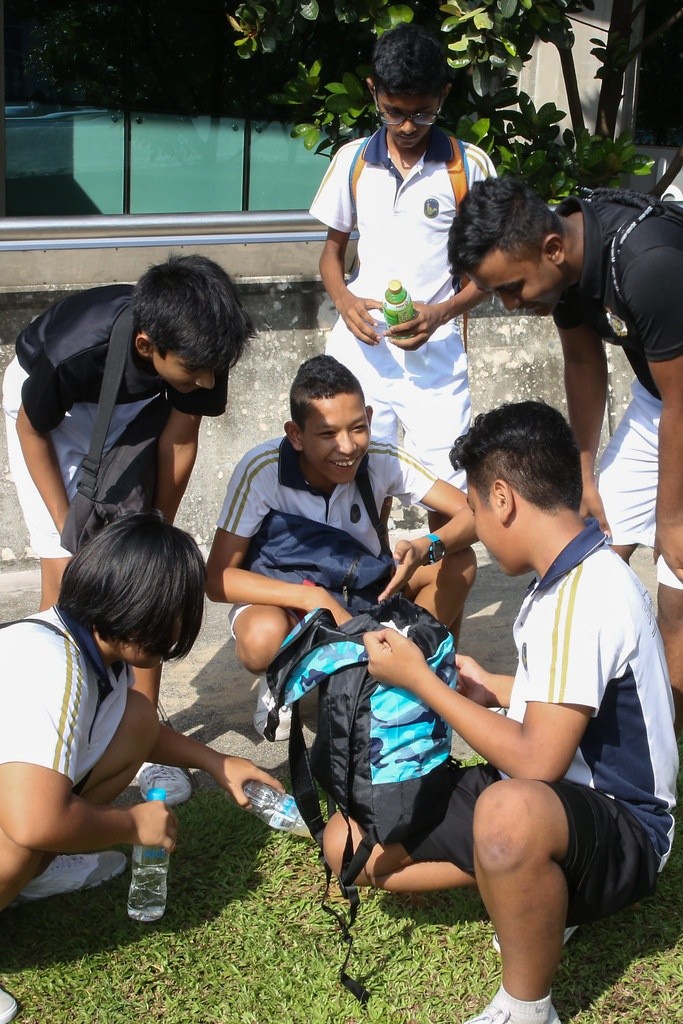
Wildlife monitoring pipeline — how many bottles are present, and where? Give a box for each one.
[127,788,172,922]
[383,279,415,341]
[224,778,317,841]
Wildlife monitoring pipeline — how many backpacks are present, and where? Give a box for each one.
[587,185,683,310]
[59,300,161,554]
[239,509,413,617]
[264,594,462,1007]
[347,136,474,354]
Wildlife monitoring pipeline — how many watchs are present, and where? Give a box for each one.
[423,533,447,566]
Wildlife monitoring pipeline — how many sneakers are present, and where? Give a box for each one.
[249,674,291,741]
[493,925,578,954]
[0,988,18,1024]
[136,761,192,806]
[463,1004,562,1024]
[20,851,128,899]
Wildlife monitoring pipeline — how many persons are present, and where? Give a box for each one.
[323,401,683,1023]
[2,254,256,803]
[309,23,499,654]
[446,169,683,724]
[0,510,286,1024]
[205,353,478,742]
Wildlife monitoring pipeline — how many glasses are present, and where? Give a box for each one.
[374,86,441,126]
